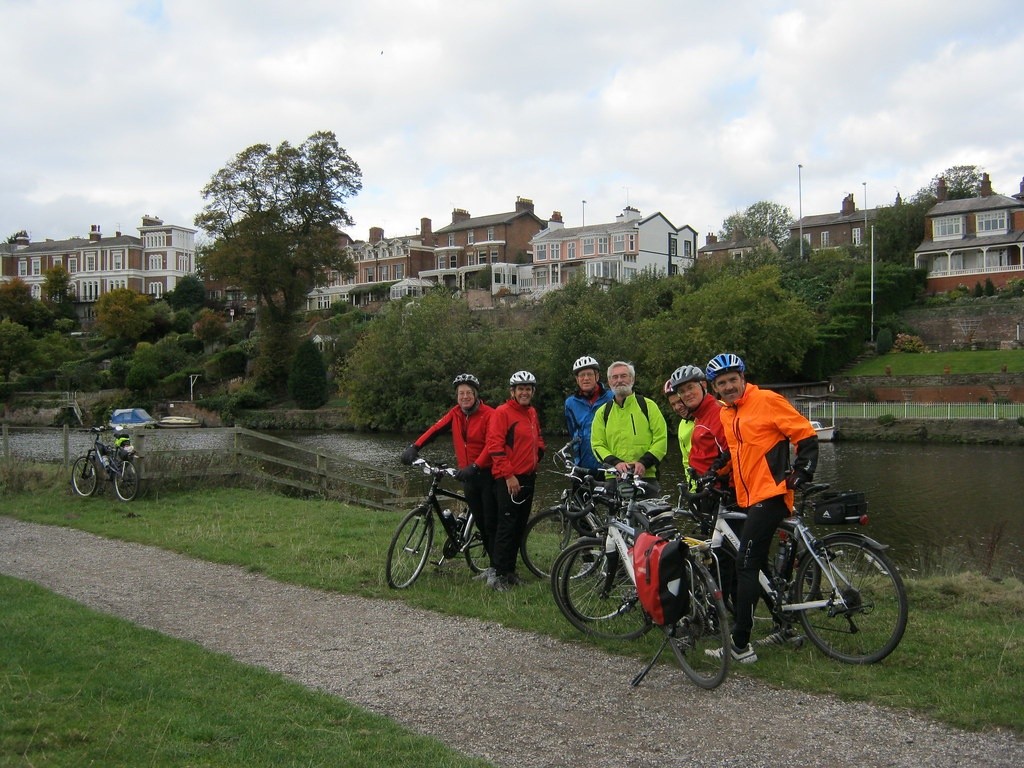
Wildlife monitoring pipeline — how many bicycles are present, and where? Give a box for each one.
[385,446,492,591]
[520,435,910,690]
[72,425,144,503]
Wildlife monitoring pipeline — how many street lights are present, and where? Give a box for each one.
[870,225,876,344]
[861,181,867,260]
[797,164,804,262]
[581,199,588,228]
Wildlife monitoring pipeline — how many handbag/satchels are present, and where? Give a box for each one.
[627,526,690,626]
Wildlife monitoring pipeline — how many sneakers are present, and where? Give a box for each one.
[704,634,758,663]
[756,628,810,650]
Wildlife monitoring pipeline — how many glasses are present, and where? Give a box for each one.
[576,373,596,377]
[457,391,475,395]
[679,383,701,395]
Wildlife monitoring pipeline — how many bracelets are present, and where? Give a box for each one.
[471,463,480,471]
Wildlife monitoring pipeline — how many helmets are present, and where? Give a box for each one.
[572,356,600,372]
[510,370,537,386]
[664,378,675,396]
[706,353,746,382]
[452,373,480,391]
[670,365,705,389]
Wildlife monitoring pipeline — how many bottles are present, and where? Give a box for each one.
[443,509,455,530]
[773,540,786,575]
[627,547,633,568]
[456,507,467,532]
[101,454,109,468]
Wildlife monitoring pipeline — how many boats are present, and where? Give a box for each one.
[109,408,160,430]
[155,415,202,429]
[809,419,836,443]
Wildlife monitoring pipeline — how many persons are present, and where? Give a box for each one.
[485,370,544,588]
[589,361,667,517]
[561,355,616,574]
[669,365,759,633]
[398,374,500,588]
[703,353,820,664]
[663,379,702,496]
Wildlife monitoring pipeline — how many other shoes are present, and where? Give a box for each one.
[471,567,527,591]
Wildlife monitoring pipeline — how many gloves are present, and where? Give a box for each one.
[785,469,809,489]
[455,463,482,483]
[400,445,421,465]
